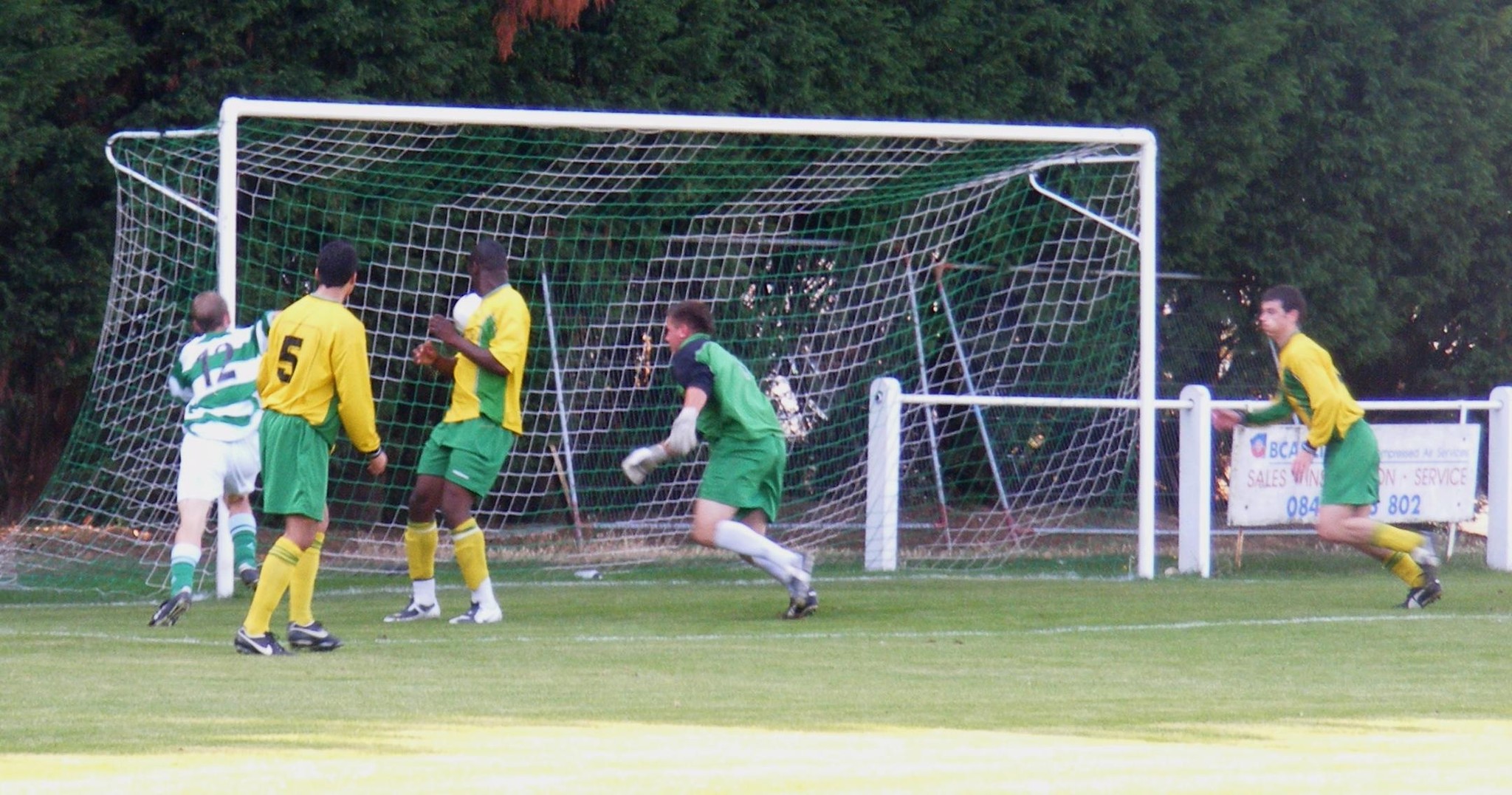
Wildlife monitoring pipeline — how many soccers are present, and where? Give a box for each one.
[453,292,482,333]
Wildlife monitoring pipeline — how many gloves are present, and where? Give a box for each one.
[665,407,699,458]
[622,444,670,485]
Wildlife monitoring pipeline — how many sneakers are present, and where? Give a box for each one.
[383,595,440,622]
[147,585,194,628]
[1410,531,1443,593]
[1391,578,1443,609]
[449,600,503,625]
[790,551,815,607]
[287,619,346,651]
[779,587,818,618]
[234,625,297,656]
[239,563,260,587]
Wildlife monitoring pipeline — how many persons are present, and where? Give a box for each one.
[622,299,818,620]
[1211,284,1443,610]
[382,240,533,625]
[234,242,387,656]
[148,292,285,628]
